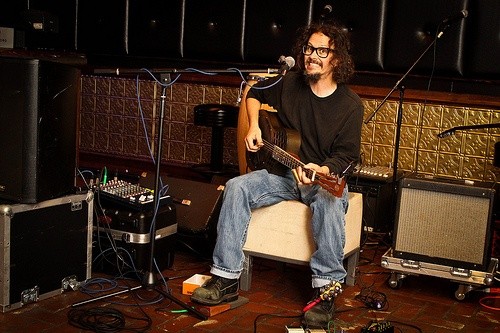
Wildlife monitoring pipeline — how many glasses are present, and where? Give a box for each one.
[302,44,333,58]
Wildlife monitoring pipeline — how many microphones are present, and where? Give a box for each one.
[319,5,332,21]
[280,56,295,78]
[443,10,468,22]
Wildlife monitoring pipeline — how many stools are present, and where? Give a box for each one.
[238,192,362,292]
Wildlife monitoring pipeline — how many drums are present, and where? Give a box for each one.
[237,73,280,176]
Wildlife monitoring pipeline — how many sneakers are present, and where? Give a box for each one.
[302,295,335,329]
[190,275,240,306]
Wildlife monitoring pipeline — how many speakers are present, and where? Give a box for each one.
[391,171,498,272]
[0,56,71,204]
[135,172,225,255]
[348,180,392,232]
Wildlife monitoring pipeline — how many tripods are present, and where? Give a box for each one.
[71,69,281,321]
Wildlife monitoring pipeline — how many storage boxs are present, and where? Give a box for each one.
[182,273,212,295]
[0,189,95,312]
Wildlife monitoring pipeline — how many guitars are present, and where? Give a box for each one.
[245,109,346,198]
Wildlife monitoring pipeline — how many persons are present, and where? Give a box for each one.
[189,24,364,328]
